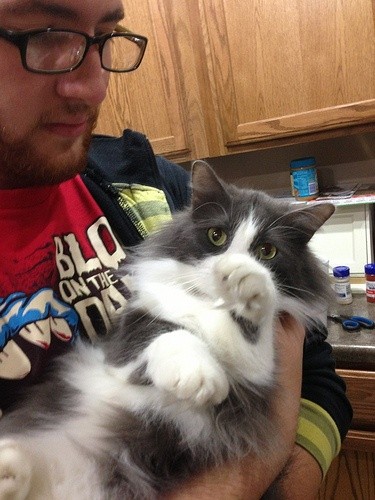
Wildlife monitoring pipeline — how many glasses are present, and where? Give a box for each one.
[0,24,148,74]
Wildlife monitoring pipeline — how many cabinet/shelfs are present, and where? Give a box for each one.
[92,0,375,164]
[313,294,375,500]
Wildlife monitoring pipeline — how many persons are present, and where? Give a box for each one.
[0,0,354,500]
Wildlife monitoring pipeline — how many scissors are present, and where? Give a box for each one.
[328,313,374,331]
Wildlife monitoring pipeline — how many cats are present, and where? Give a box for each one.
[0,160,339,500]
